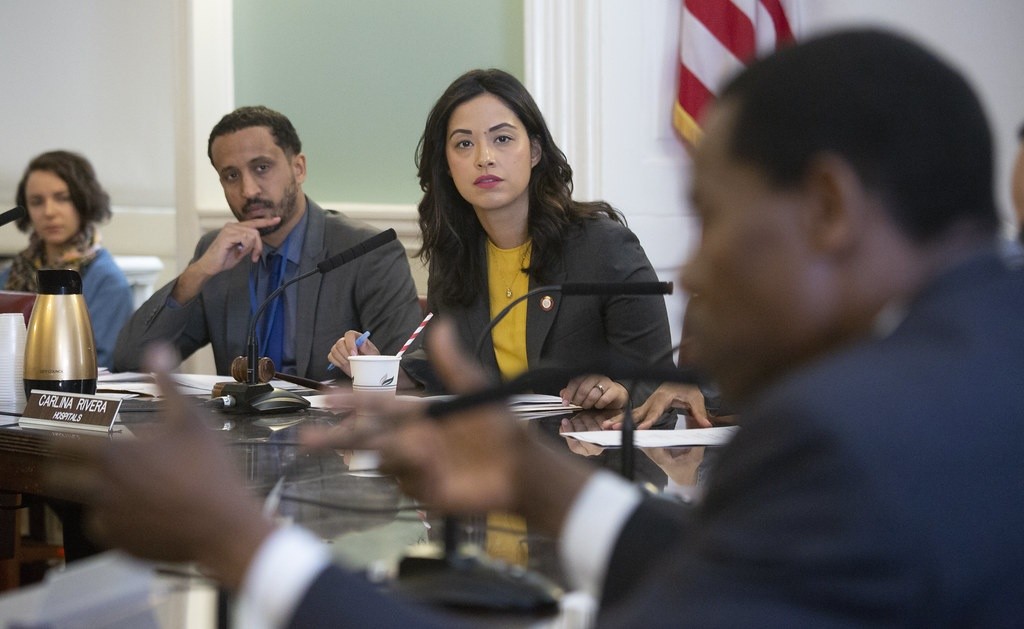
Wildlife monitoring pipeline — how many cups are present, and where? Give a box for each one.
[347,355,402,398]
[0,312,29,412]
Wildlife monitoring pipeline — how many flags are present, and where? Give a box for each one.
[672,0,799,157]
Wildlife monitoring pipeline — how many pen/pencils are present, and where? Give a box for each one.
[237,244,243,251]
[328,330,370,371]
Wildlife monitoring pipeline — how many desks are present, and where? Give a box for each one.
[0,370,690,629]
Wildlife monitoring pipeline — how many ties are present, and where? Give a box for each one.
[250,251,288,374]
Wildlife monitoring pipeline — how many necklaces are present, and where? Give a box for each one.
[491,244,528,298]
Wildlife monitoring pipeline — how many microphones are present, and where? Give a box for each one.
[0,206,27,226]
[222,227,673,613]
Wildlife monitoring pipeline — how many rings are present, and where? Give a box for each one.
[594,384,604,396]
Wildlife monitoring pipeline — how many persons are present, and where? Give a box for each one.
[640,446,706,505]
[108,107,424,388]
[560,412,617,456]
[0,152,133,368]
[51,32,1024,628]
[226,416,401,544]
[328,70,675,409]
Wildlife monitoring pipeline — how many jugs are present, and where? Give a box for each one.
[24,269,98,404]
